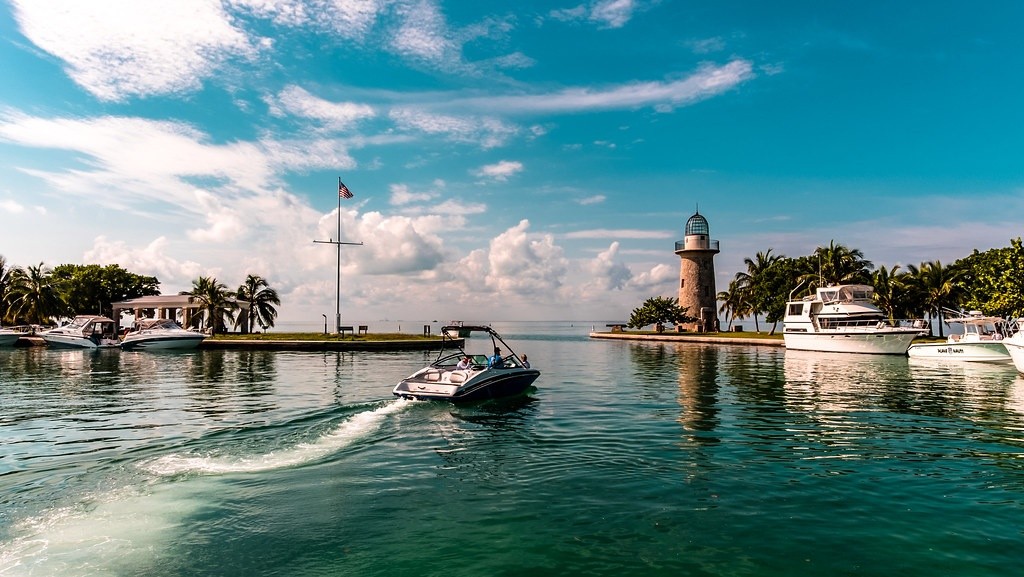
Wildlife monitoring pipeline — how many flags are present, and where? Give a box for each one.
[340,181,354,199]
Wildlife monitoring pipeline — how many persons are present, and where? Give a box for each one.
[520,354,530,369]
[488,347,504,369]
[714,319,718,334]
[992,330,1003,340]
[455,356,475,370]
[876,318,886,328]
[702,318,708,333]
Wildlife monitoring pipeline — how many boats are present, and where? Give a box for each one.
[41,314,122,350]
[902,303,1014,363]
[1000,318,1024,373]
[781,251,930,354]
[390,324,539,410]
[125,317,212,356]
[0,324,46,350]
